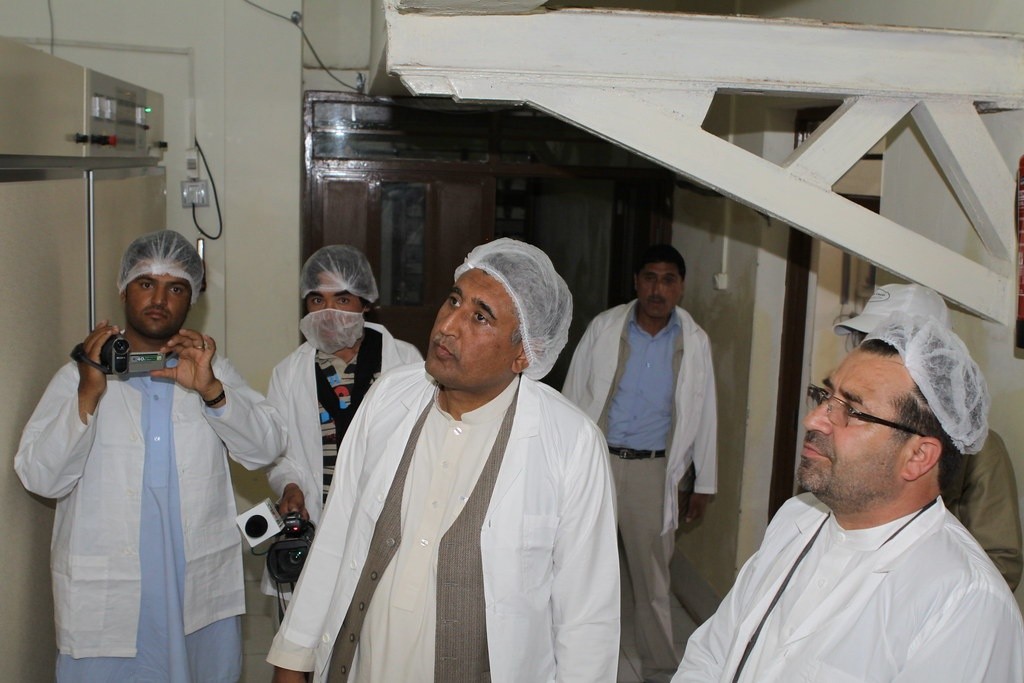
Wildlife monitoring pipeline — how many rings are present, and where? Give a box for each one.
[193,345,203,350]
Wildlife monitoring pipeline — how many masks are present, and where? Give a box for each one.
[299,308,364,355]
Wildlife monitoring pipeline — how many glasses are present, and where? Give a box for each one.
[806,384,927,437]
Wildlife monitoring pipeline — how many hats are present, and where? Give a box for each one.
[833,283,953,335]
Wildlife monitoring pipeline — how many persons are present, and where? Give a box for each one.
[259,247,429,639]
[267,240,622,683]
[561,240,716,683]
[12,231,289,683]
[670,282,1024,683]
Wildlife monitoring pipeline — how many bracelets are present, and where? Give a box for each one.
[204,391,226,407]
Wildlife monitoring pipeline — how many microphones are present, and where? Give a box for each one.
[235,497,285,548]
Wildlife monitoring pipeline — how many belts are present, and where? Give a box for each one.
[608,445,666,459]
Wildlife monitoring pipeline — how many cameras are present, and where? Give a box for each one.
[99,333,166,376]
